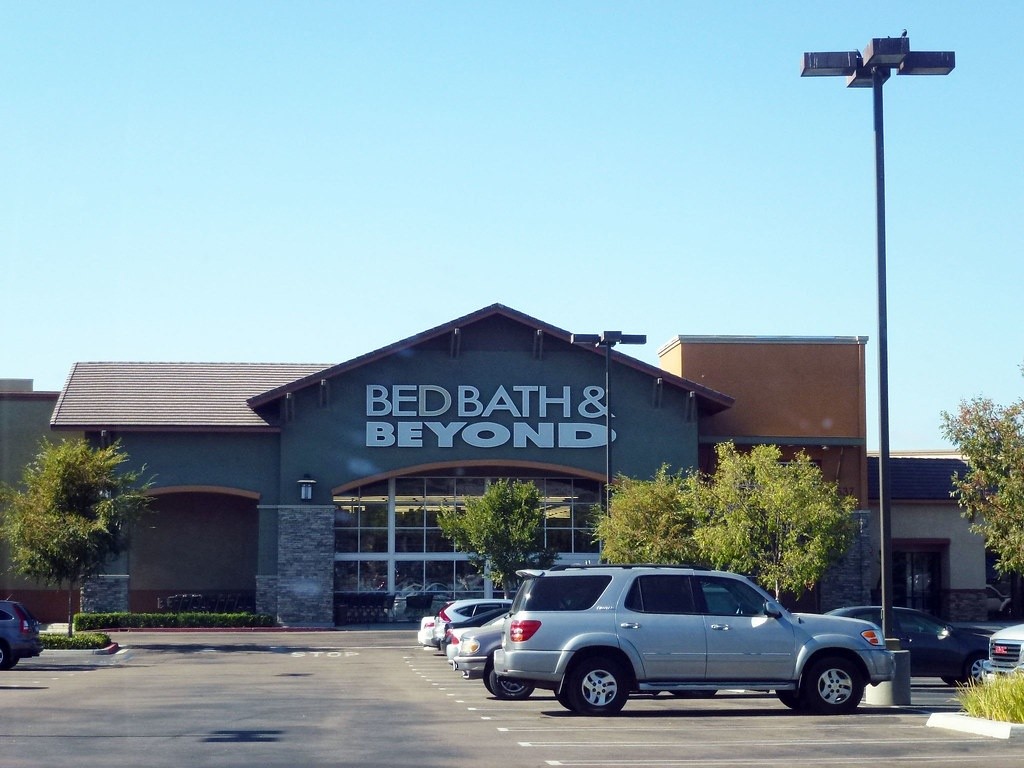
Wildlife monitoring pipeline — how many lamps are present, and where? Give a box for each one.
[799,51,859,77]
[861,37,910,68]
[845,65,890,88]
[895,50,956,75]
[296,474,318,501]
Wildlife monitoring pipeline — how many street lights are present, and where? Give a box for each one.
[794,27,964,650]
[570,329,649,564]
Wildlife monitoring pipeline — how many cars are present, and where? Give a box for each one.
[452,627,535,700]
[982,623,1024,690]
[438,607,510,655]
[418,617,443,653]
[444,612,510,665]
[817,605,995,692]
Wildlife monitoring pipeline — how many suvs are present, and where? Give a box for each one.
[0,593,44,674]
[426,596,515,649]
[490,563,897,714]
